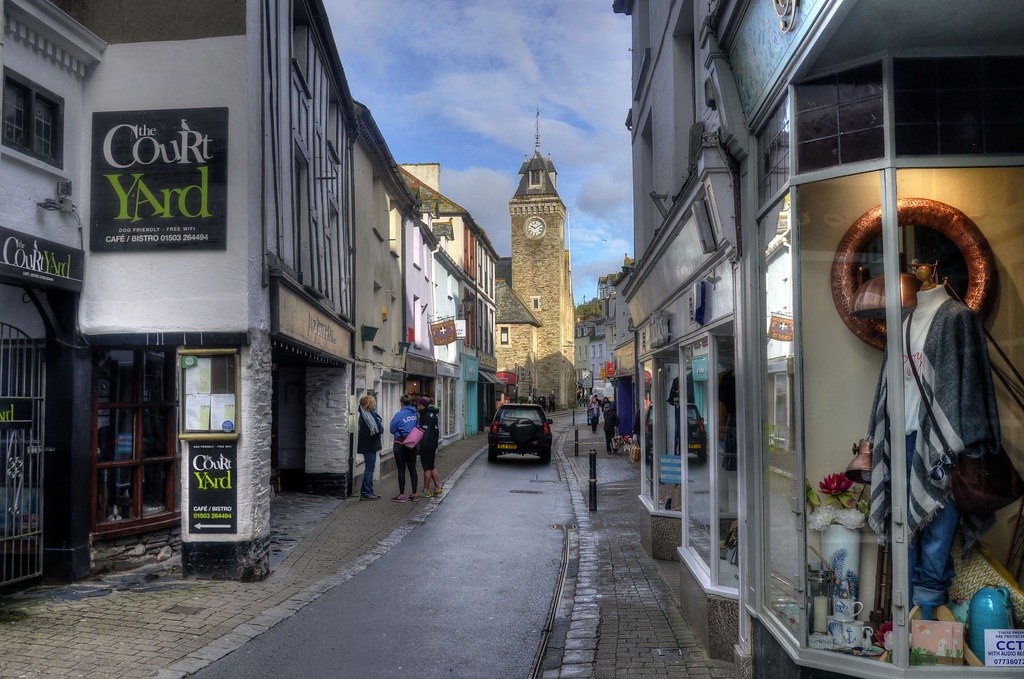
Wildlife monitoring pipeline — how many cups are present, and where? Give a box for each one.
[832,595,863,622]
[843,620,872,647]
[827,616,842,637]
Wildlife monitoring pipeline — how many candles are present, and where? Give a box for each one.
[814,596,827,632]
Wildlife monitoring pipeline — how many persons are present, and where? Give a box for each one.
[717,360,739,512]
[357,396,384,501]
[868,283,1000,621]
[537,390,641,454]
[416,397,442,497]
[390,394,420,503]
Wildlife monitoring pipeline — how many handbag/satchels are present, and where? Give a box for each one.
[720,525,738,567]
[665,498,672,510]
[612,426,624,448]
[941,439,1024,511]
[402,426,425,448]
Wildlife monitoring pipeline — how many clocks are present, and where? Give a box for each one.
[527,221,543,236]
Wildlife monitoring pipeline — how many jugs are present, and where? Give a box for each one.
[969,584,1013,663]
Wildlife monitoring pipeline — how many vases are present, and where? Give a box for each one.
[832,595,863,623]
[820,525,860,600]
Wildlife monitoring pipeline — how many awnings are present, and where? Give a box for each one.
[480,372,505,386]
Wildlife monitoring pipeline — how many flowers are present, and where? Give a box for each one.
[788,473,869,531]
[833,579,852,599]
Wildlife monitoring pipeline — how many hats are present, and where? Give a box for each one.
[605,404,611,408]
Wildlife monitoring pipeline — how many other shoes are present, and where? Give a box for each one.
[408,493,418,502]
[391,494,405,502]
[418,489,433,498]
[432,488,444,496]
[360,492,381,501]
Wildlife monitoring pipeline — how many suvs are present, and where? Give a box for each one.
[645,399,708,465]
[488,402,553,463]
